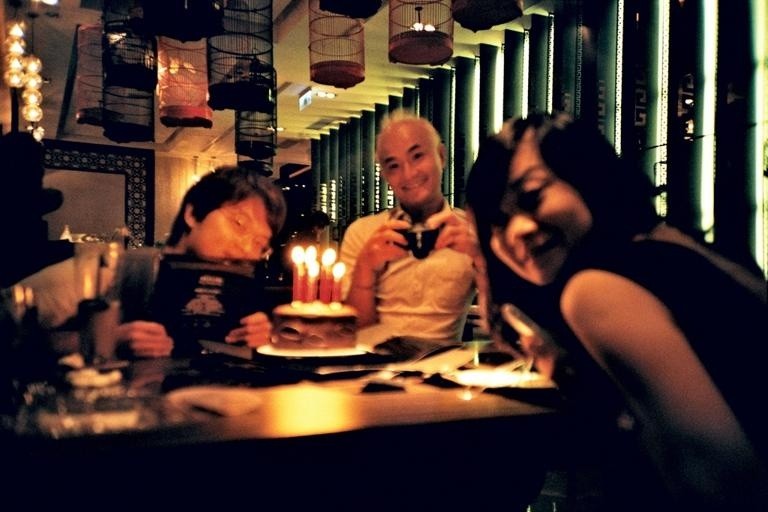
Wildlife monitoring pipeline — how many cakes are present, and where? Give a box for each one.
[269,301,357,351]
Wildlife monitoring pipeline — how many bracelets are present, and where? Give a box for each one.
[470,262,488,276]
[353,283,374,290]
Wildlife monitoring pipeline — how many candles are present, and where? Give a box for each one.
[289,242,349,303]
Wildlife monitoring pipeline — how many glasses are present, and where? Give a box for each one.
[217,207,273,262]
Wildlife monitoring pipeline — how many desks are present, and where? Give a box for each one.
[1,348,567,451]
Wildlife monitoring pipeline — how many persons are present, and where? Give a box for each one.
[456,109,768,512]
[80,165,287,358]
[309,107,491,353]
